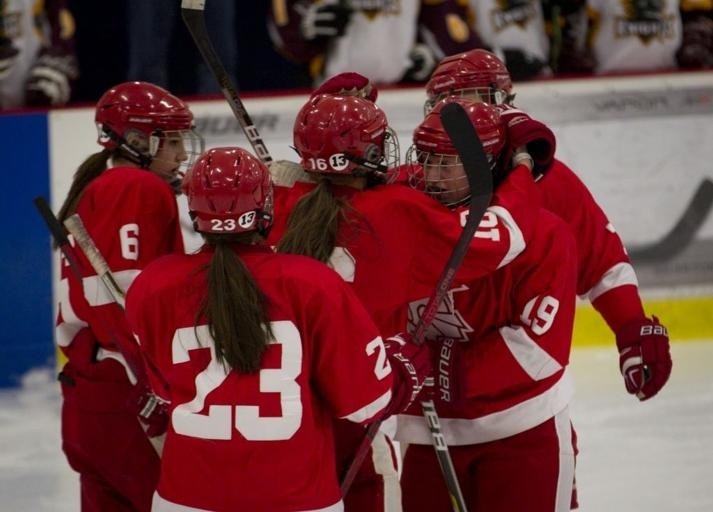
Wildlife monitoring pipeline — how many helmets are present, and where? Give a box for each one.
[405,94,508,214]
[289,92,402,191]
[420,49,515,118]
[178,146,277,246]
[93,80,206,196]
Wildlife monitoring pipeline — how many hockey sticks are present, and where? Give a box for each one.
[624,181,713,266]
[269,159,469,512]
[181,0,273,170]
[337,104,497,500]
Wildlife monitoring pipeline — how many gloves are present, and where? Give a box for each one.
[611,314,673,403]
[311,72,379,102]
[383,332,435,418]
[495,103,556,183]
[125,378,169,439]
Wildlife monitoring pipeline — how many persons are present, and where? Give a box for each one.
[375,98,586,511]
[112,148,433,511]
[49,81,208,511]
[414,41,673,511]
[305,71,431,191]
[1,1,713,108]
[262,96,560,511]
